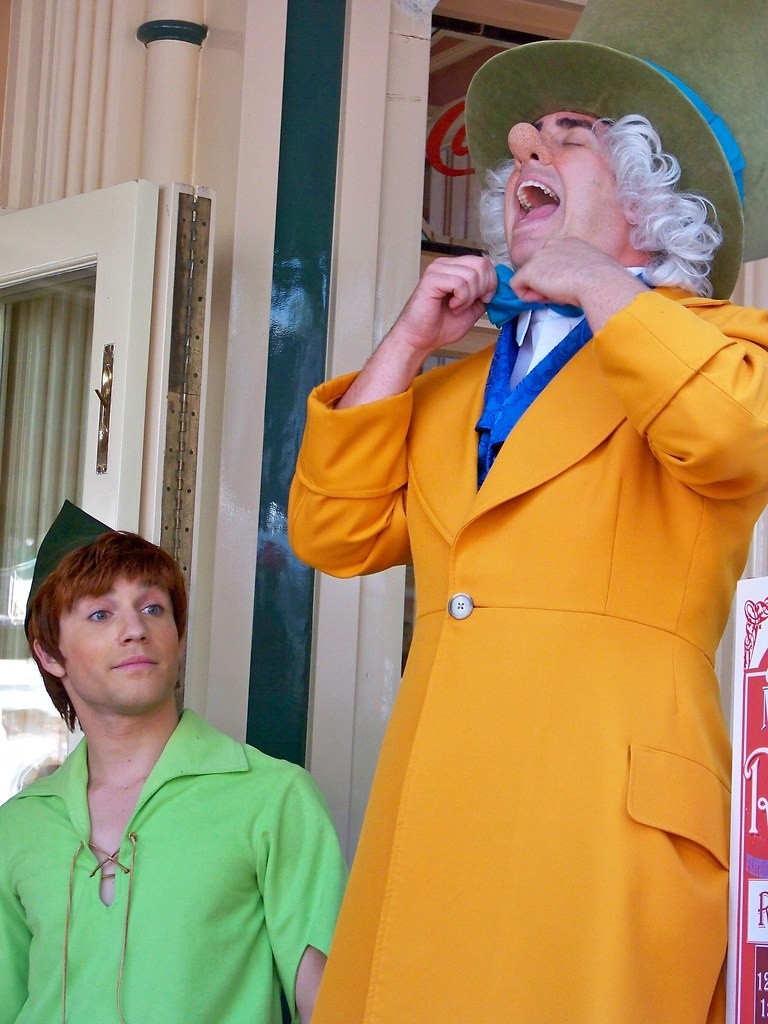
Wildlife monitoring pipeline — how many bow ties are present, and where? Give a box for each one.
[482,264,583,329]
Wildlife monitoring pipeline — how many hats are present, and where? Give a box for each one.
[24,499,119,613]
[464,40,746,300]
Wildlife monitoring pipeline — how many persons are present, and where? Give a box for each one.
[0,500,349,1024]
[285,1,768,1023]
